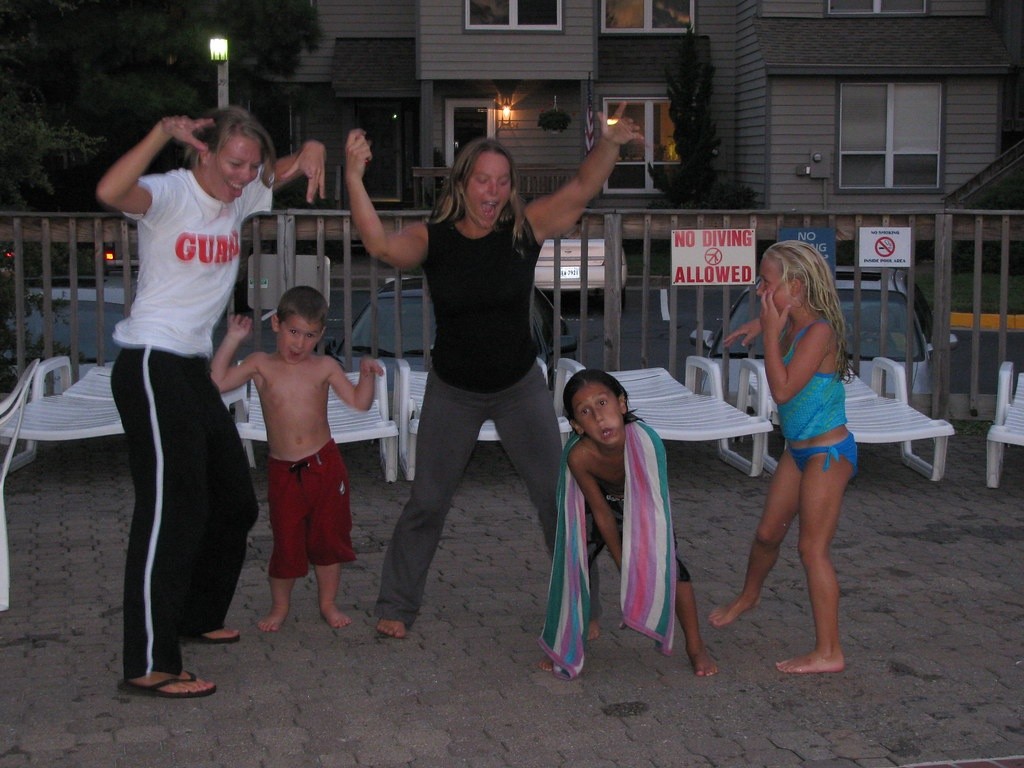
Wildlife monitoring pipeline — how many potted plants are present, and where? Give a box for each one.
[538,95,571,132]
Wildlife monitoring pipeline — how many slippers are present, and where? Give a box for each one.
[193,632,240,645]
[121,671,216,698]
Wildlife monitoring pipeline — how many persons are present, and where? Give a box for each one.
[709,240,858,674]
[95,105,324,698]
[343,102,645,648]
[524,368,718,676]
[211,285,385,636]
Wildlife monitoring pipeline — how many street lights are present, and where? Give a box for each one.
[210,39,230,110]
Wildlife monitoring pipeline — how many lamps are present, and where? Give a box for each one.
[498,96,514,129]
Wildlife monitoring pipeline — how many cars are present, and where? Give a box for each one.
[532,238,630,317]
[312,273,579,367]
[2,269,137,379]
[688,265,959,396]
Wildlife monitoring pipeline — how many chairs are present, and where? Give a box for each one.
[0,357,956,483]
[986,361,1024,488]
